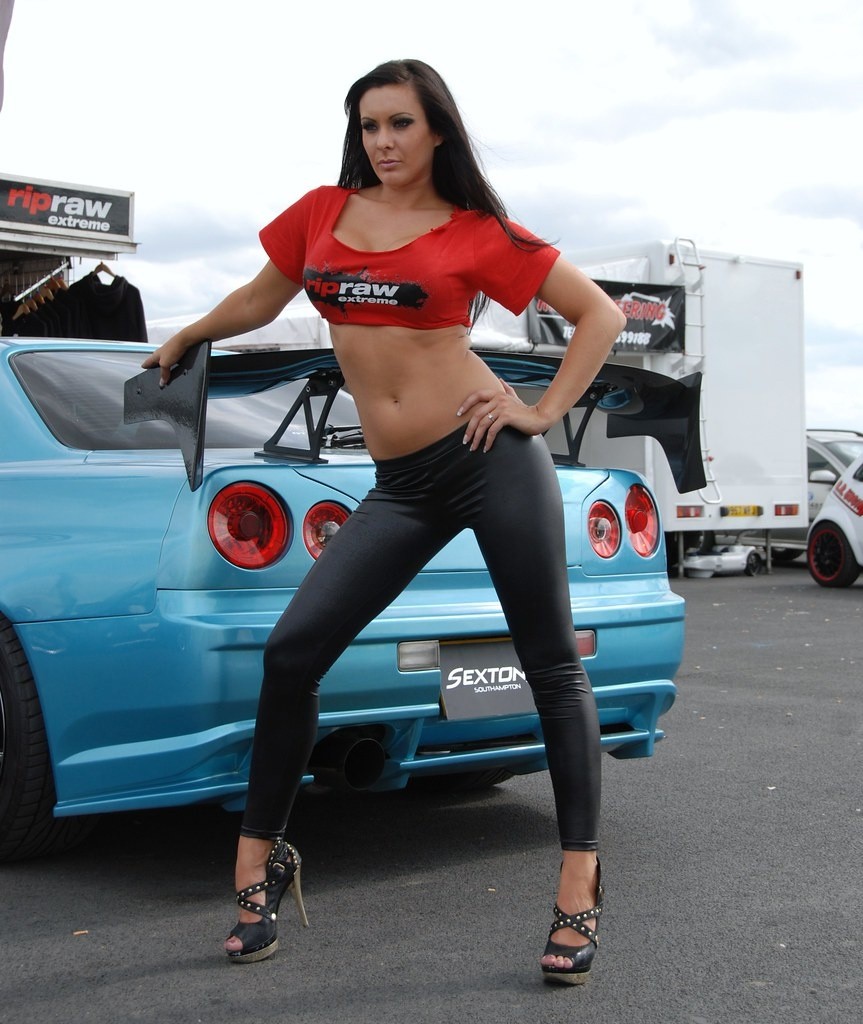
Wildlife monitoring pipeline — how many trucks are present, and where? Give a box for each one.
[463,239,812,577]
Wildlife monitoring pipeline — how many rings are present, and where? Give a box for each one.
[487,413,495,423]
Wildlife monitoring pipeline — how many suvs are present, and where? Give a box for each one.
[702,428,863,565]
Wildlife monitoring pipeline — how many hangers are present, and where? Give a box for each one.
[0,257,117,322]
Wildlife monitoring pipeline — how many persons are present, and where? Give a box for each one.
[139,55,632,986]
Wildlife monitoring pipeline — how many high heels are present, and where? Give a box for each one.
[540,856,604,990]
[225,839,311,962]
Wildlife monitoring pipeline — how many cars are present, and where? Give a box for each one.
[797,457,863,591]
[2,331,691,873]
